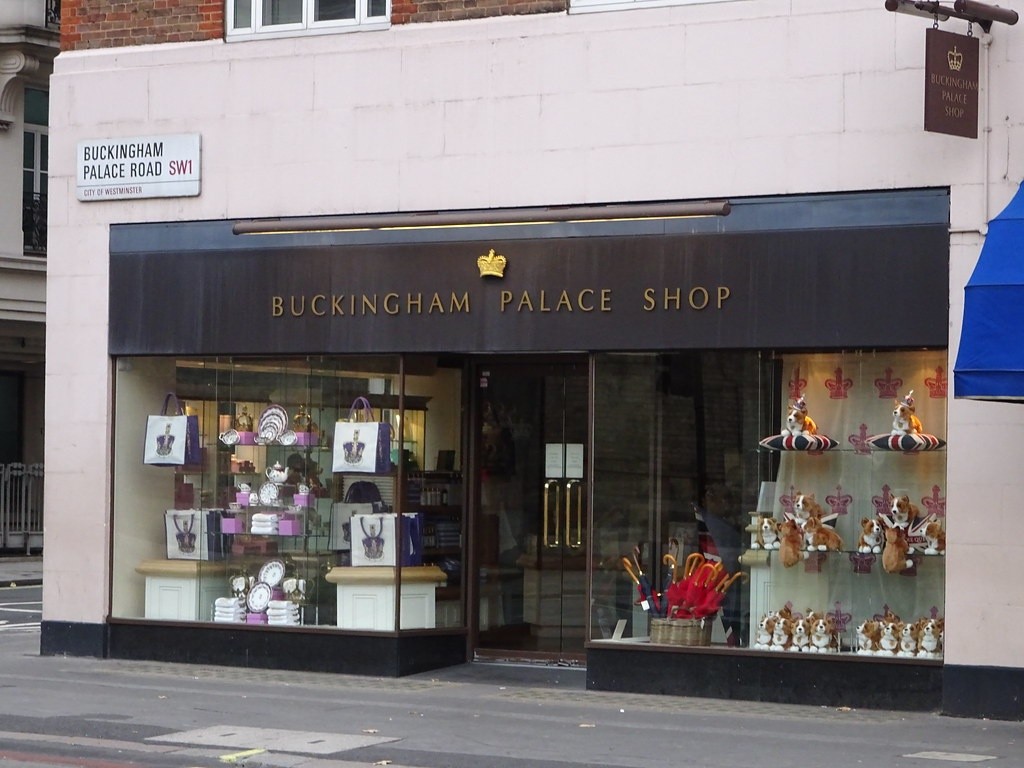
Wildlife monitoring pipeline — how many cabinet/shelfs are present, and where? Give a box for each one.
[324,566,448,631]
[516,553,630,638]
[176,390,434,603]
[134,552,293,620]
[340,466,463,603]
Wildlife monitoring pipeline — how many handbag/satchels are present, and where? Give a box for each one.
[163,506,235,561]
[142,391,204,468]
[326,482,423,566]
[332,397,393,476]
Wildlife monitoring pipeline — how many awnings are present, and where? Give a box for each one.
[953,183,1024,404]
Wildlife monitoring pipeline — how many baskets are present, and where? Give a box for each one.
[649,606,717,647]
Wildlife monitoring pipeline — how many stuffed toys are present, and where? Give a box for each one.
[781,404,817,436]
[757,609,944,660]
[759,491,840,567]
[858,495,945,572]
[892,398,922,436]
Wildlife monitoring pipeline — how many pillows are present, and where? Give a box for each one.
[782,511,839,550]
[863,434,948,451]
[759,434,841,452]
[879,512,938,553]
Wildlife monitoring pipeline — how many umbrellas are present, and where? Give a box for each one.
[664,553,749,621]
[622,544,661,618]
